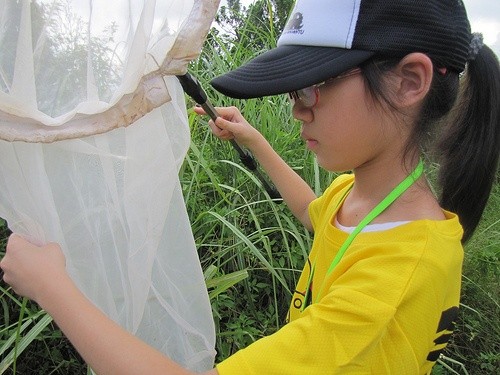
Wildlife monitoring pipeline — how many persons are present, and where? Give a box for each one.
[0,0,500,373]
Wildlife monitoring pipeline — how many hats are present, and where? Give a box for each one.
[206,0,471,100]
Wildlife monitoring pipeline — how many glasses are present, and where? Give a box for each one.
[286,60,448,108]
